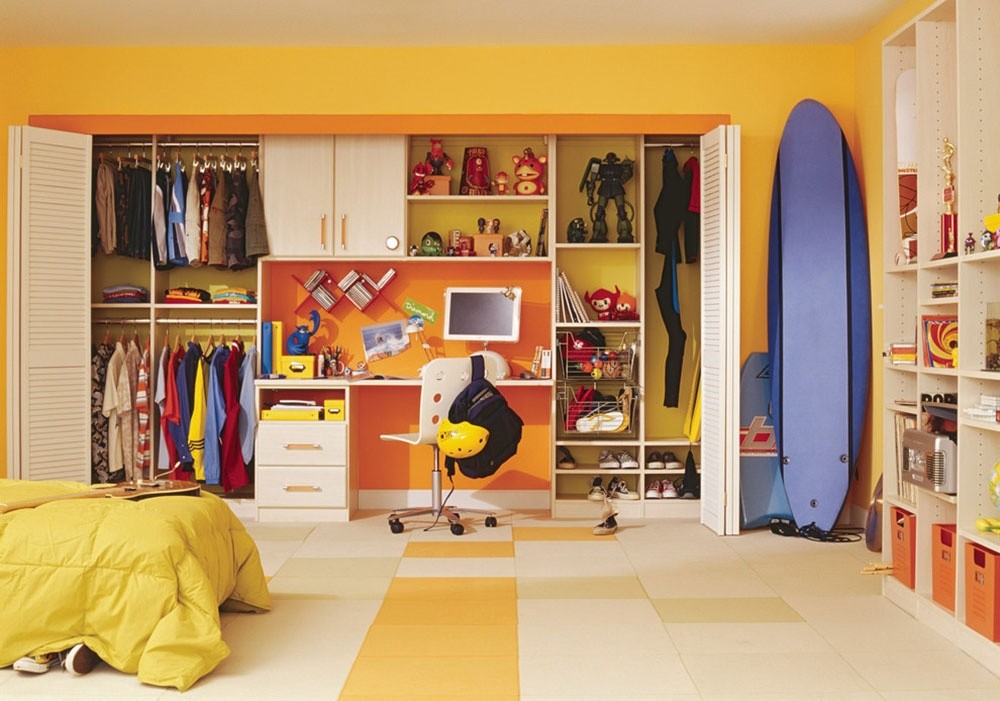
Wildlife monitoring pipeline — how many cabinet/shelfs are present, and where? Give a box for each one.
[880,0,1000,673]
[254,254,555,525]
[409,134,553,262]
[4,127,262,499]
[550,123,742,537]
[258,133,409,257]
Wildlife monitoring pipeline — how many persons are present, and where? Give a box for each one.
[586,152,634,243]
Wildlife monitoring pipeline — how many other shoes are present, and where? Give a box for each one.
[616,449,639,469]
[598,449,621,468]
[662,450,683,469]
[674,476,698,499]
[646,450,664,469]
[559,447,576,469]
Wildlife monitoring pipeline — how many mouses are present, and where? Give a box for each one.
[520,369,536,380]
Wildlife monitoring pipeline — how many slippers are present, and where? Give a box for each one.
[576,411,629,433]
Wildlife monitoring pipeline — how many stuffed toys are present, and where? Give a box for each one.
[583,285,621,321]
[615,289,636,314]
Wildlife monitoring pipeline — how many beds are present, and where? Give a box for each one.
[0,480,273,693]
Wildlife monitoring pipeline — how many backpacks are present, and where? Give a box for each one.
[449,355,523,480]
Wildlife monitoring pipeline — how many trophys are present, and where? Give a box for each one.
[931,136,957,259]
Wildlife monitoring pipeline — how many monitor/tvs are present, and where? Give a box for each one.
[444,286,522,342]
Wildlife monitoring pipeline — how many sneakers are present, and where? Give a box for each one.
[662,480,678,498]
[607,476,639,500]
[645,479,663,497]
[14,651,59,673]
[59,643,104,676]
[591,512,619,535]
[587,475,608,501]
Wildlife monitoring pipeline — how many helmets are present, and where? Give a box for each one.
[435,418,489,459]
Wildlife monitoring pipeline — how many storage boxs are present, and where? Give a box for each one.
[425,175,450,195]
[474,234,505,257]
[324,400,344,420]
[965,542,1000,642]
[282,355,316,379]
[932,522,957,612]
[890,507,916,589]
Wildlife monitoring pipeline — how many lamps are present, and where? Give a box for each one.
[405,314,436,362]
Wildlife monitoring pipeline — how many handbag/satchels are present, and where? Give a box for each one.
[564,384,594,429]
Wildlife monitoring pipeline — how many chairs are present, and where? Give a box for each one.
[379,354,498,536]
